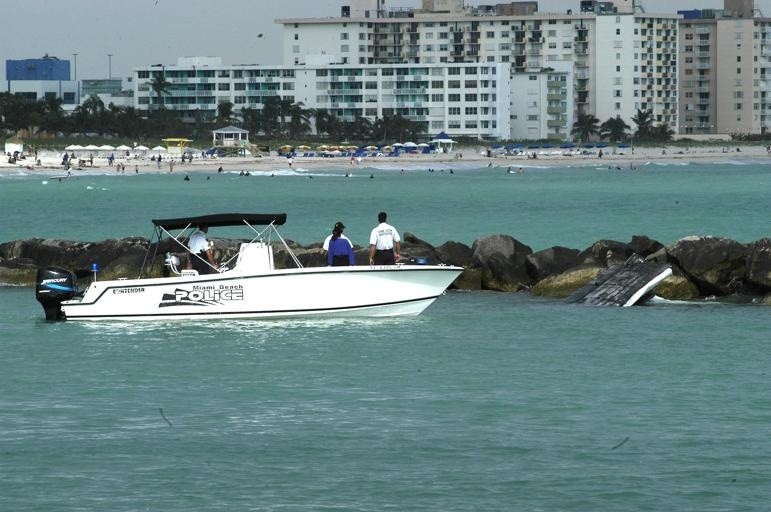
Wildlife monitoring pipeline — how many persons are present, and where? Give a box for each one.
[345,157,455,179]
[488,163,523,174]
[9,148,139,179]
[186,223,218,274]
[287,157,294,166]
[369,212,400,265]
[151,152,250,181]
[322,222,353,259]
[326,227,354,266]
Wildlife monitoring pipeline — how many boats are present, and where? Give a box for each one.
[36,214,467,321]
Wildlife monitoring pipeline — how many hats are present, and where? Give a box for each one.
[333,222,346,234]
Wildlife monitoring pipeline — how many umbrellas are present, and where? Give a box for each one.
[278,142,630,156]
